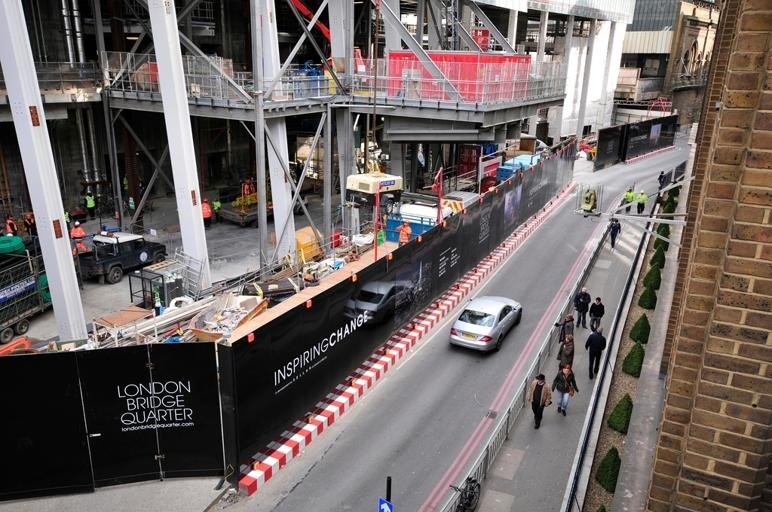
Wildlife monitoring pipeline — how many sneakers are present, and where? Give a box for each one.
[557,406,566,416]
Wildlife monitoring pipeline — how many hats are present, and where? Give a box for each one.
[536,374,545,380]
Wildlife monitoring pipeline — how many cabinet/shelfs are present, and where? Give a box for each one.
[128,259,188,312]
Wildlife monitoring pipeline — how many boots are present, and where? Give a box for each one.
[535,421,540,429]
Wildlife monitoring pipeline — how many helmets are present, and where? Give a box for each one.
[74,221,80,226]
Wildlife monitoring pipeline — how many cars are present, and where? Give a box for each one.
[449,295,522,352]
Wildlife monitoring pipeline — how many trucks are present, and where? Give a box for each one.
[0,236,52,344]
[216,160,309,227]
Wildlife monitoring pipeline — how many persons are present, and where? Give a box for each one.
[624,188,635,212]
[551,365,581,413]
[212,198,222,221]
[0,208,90,257]
[244,179,254,196]
[585,327,611,379]
[395,219,413,248]
[526,372,552,429]
[84,190,98,220]
[201,198,214,228]
[635,190,649,214]
[553,314,574,341]
[572,286,592,329]
[587,295,604,333]
[557,332,577,375]
[608,218,621,248]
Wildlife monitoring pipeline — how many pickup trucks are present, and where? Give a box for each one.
[73,231,168,284]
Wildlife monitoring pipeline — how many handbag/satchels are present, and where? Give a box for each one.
[569,386,574,397]
